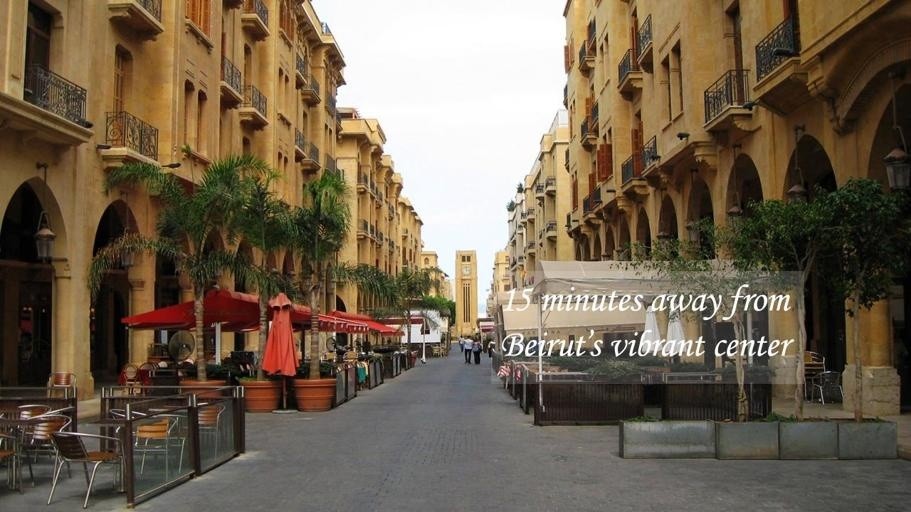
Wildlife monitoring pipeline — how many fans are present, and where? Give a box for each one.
[167,330,194,361]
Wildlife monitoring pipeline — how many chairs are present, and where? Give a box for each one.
[801,351,827,400]
[120,360,198,397]
[811,371,843,405]
[46,372,77,399]
[432,346,446,359]
[1,399,232,511]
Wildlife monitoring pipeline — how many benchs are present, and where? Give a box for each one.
[491,344,643,426]
[298,350,411,400]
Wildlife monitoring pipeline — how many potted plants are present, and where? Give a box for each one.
[393,265,448,368]
[87,153,268,398]
[288,169,353,413]
[235,169,284,415]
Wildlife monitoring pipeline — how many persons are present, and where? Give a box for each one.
[488,339,495,358]
[464,335,474,364]
[473,338,483,364]
[458,335,465,352]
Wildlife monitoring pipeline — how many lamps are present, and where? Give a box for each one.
[35,162,56,262]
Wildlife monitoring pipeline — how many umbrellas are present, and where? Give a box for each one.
[664,302,688,362]
[641,304,664,356]
[262,291,301,414]
